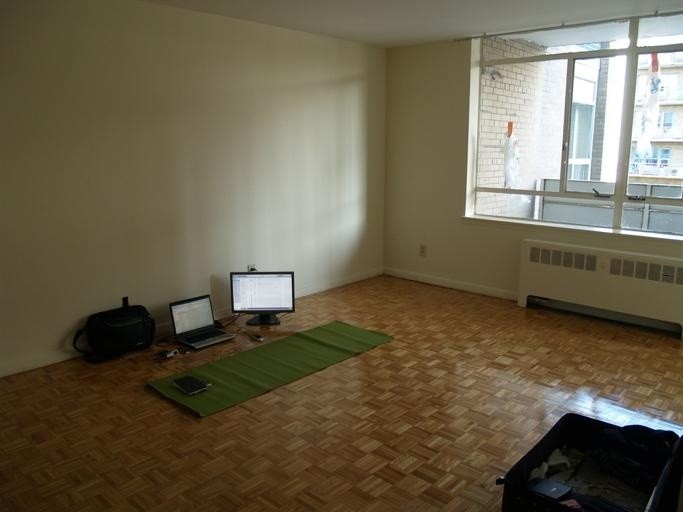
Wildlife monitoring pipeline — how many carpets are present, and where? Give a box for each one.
[145,320,393,416]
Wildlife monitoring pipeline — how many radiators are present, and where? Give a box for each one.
[518,238,683,340]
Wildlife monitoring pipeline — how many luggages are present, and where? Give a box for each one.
[496,412,682,511]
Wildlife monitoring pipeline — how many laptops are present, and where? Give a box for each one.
[169,294,234,350]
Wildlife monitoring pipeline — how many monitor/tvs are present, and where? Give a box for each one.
[230,270,295,324]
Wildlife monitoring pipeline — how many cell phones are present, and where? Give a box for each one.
[252,334,264,342]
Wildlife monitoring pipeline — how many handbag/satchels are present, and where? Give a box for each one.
[84,305,155,364]
